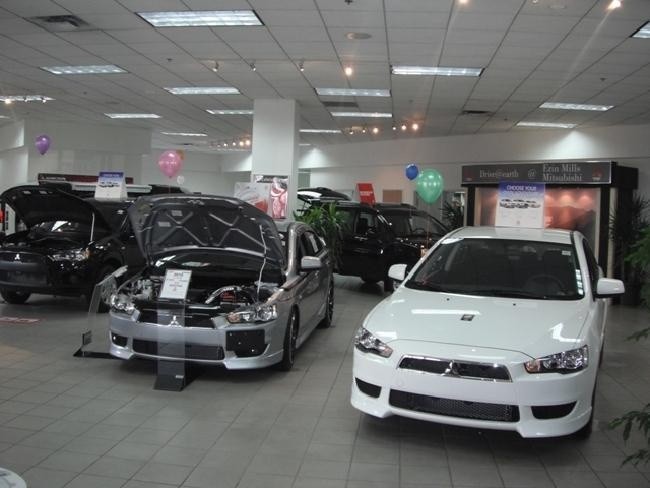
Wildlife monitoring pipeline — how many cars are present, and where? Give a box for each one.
[0,181,146,312]
[501,197,539,211]
[348,223,628,444]
[108,193,336,373]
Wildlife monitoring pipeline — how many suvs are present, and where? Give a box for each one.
[295,187,452,295]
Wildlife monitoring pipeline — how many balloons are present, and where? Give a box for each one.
[415,168,444,204]
[36,134,52,156]
[159,151,181,177]
[405,162,417,180]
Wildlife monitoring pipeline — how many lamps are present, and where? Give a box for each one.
[208,138,251,150]
[210,60,305,73]
[349,123,419,135]
[4,97,48,104]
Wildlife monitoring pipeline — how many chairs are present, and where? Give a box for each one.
[357,218,369,234]
[538,251,571,289]
